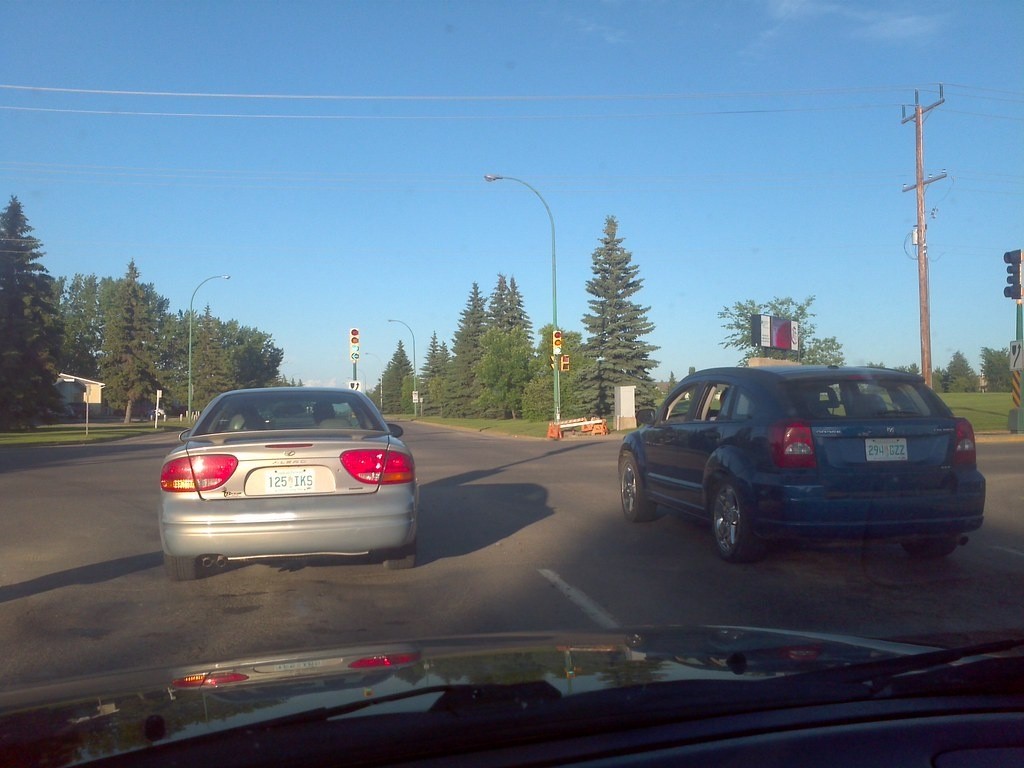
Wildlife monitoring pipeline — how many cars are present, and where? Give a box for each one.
[618,364,987,563]
[159,386,419,581]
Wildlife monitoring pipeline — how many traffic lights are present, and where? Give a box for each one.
[1003,249,1021,298]
[350,328,360,362]
[552,331,562,354]
[562,355,570,371]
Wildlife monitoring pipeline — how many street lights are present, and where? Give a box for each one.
[188,275,231,423]
[485,176,561,422]
[388,319,417,415]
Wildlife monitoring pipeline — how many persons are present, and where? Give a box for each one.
[312,400,335,424]
